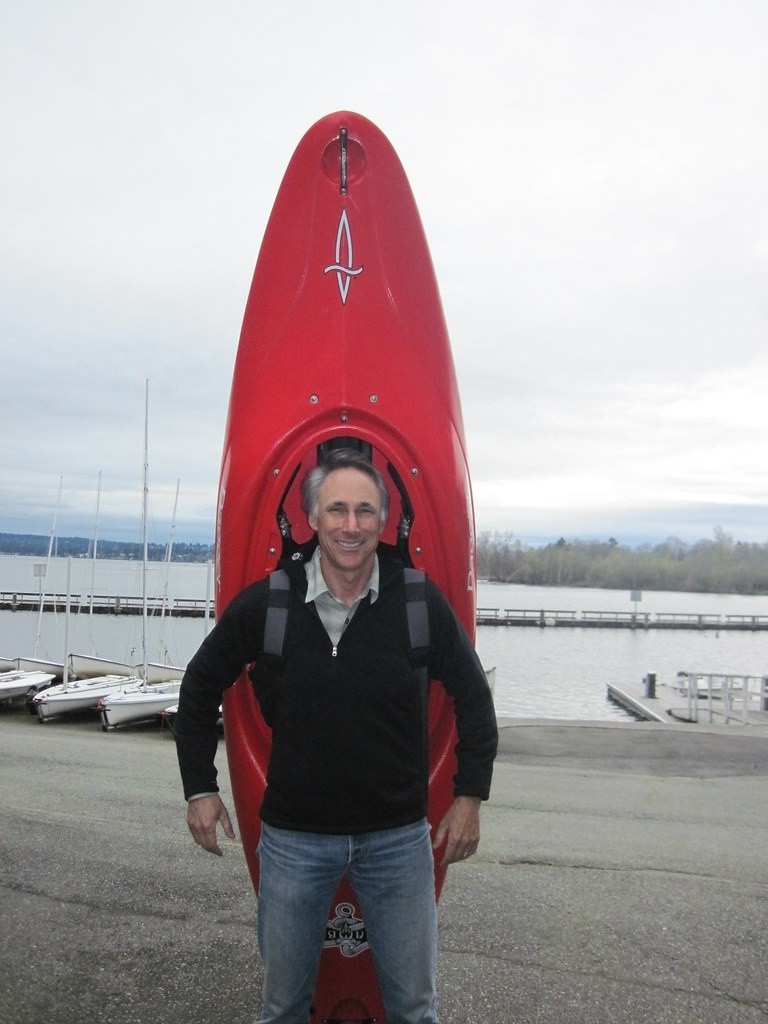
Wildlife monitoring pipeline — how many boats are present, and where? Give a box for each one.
[211,110,475,1024]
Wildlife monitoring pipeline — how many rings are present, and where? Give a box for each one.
[464,852,468,857]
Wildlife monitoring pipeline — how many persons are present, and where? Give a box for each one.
[173,447,498,1024]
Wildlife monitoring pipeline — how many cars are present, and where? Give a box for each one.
[0,656,14,672]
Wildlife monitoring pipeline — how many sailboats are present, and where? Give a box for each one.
[96,377,183,732]
[0,473,66,706]
[12,494,68,681]
[137,479,187,685]
[68,470,134,677]
[33,556,143,722]
[163,551,224,738]
